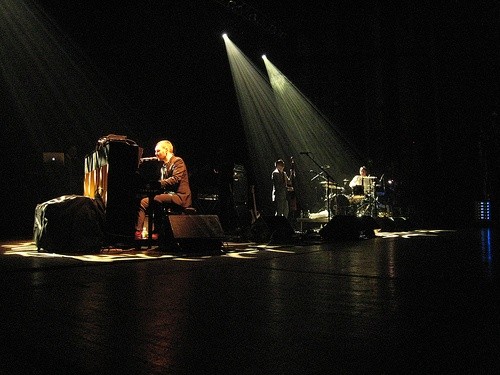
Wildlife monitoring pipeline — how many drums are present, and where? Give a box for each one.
[330,195,364,215]
[352,184,365,200]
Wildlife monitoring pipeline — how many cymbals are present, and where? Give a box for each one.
[320,181,337,184]
[324,184,343,189]
[359,175,377,178]
[367,184,383,187]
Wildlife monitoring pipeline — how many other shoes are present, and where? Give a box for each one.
[146,245,178,257]
[135,232,142,239]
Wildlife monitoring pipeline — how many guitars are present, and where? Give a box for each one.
[287,156,296,200]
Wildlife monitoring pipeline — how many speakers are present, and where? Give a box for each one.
[164,214,226,254]
[319,216,414,242]
[248,215,294,239]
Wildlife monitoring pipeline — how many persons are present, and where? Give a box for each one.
[271,159,294,218]
[132,140,192,241]
[349,166,369,189]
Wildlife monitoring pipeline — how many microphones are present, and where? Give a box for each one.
[300,151,311,155]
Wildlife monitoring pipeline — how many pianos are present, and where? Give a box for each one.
[83,139,166,251]
